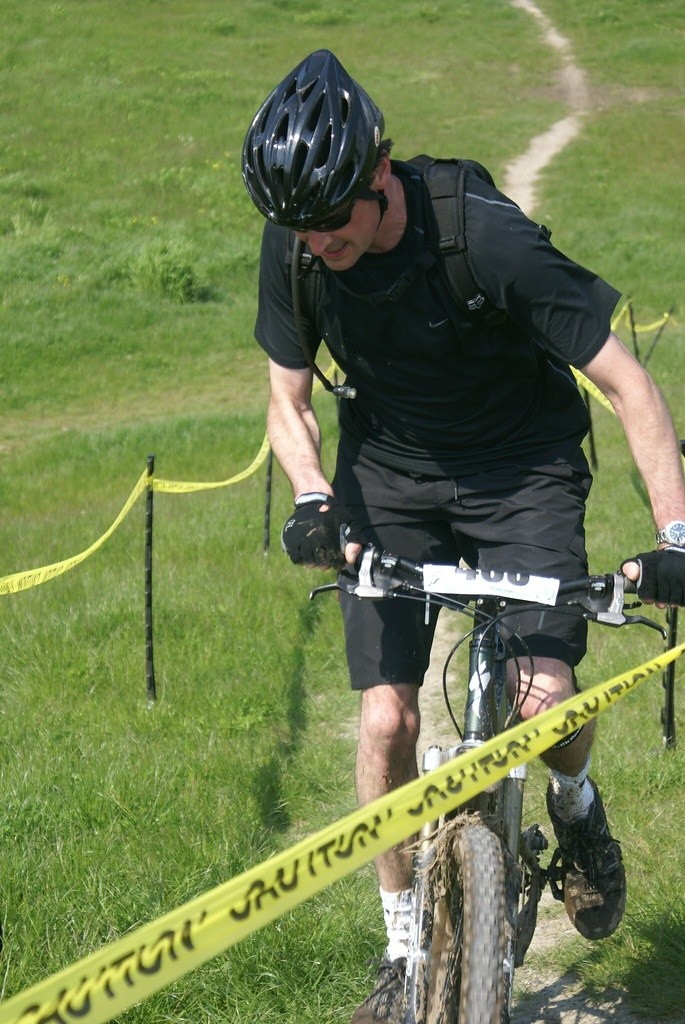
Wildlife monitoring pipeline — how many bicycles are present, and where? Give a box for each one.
[309,545,668,1023]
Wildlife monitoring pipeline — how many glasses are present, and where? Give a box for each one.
[284,181,369,233]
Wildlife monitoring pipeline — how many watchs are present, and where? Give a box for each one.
[657,520,685,549]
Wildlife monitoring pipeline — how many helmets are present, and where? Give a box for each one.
[241,50,386,230]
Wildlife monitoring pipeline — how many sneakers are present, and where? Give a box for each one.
[350,948,409,1024]
[546,774,627,940]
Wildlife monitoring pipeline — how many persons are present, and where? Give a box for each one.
[242,49,685,1024]
